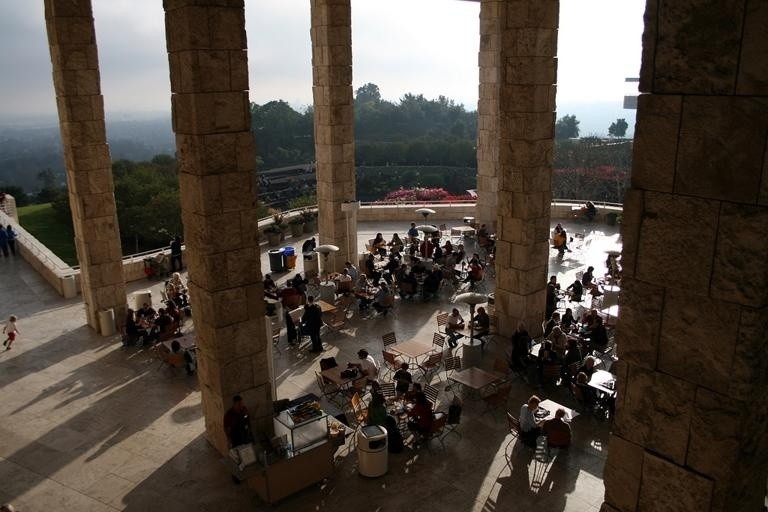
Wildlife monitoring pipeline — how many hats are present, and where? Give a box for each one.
[356,348,371,355]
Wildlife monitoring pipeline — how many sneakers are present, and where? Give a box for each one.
[3,340,13,350]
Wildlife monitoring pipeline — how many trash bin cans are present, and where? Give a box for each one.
[61,274,77,300]
[268,247,296,272]
[303,251,319,276]
[357,423,388,477]
[98,307,117,337]
[132,290,152,320]
[463,217,475,228]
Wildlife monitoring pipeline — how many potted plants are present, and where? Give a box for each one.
[259,207,318,246]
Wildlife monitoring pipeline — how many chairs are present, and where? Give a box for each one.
[123,272,199,377]
[264,222,497,355]
[315,270,617,454]
[549,223,569,259]
[571,206,591,222]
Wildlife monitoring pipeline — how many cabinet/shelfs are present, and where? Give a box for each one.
[228,400,354,500]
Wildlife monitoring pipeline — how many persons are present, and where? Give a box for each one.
[263,274,276,290]
[336,220,483,317]
[553,223,561,234]
[367,391,406,453]
[585,200,598,219]
[302,295,325,352]
[556,227,570,261]
[506,264,609,410]
[296,279,309,297]
[542,408,572,454]
[170,234,184,274]
[347,348,377,393]
[170,340,196,375]
[518,395,545,451]
[407,391,434,438]
[292,274,302,290]
[0,223,11,260]
[2,314,19,349]
[407,382,422,401]
[5,224,18,257]
[393,362,412,396]
[224,395,255,484]
[126,272,190,347]
